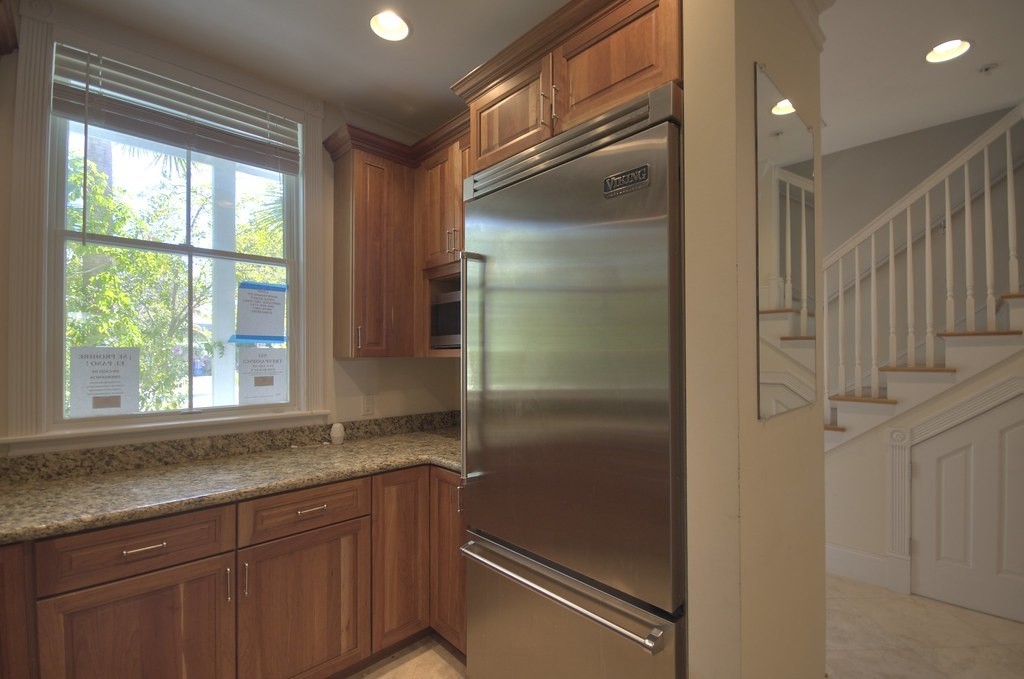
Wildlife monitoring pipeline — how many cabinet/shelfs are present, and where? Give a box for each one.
[0,465,467,679]
[322,0,680,357]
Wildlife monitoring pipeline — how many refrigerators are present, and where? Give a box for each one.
[460,82,690,678]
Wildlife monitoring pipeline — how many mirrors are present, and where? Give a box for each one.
[755,61,816,420]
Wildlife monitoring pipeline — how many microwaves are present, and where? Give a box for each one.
[429,291,460,349]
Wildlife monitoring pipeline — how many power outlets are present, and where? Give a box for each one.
[362,394,374,415]
